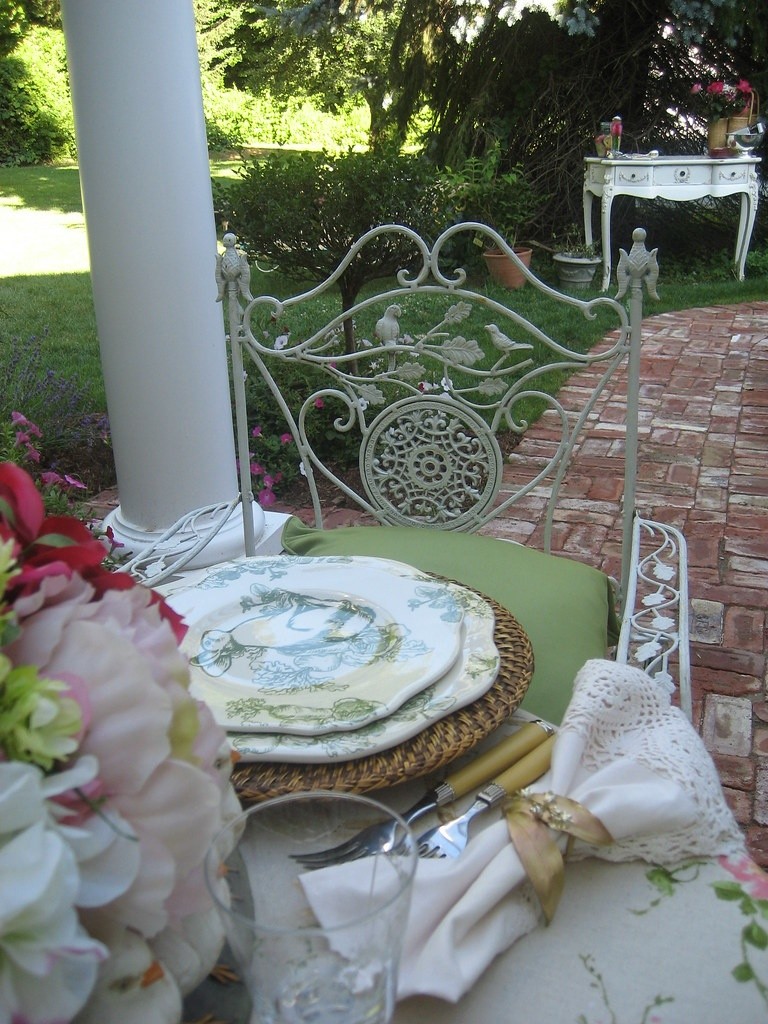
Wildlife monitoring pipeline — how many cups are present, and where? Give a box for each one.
[204,790,419,1024]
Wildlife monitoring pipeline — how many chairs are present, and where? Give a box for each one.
[123,220,693,729]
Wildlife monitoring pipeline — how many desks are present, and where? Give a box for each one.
[581,155,762,292]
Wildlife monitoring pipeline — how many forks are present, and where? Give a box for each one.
[287,719,557,871]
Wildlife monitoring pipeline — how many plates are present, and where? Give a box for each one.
[148,555,501,762]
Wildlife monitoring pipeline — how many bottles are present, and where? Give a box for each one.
[595,116,622,159]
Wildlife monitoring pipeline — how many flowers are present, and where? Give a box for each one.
[691,78,758,122]
[0,458,250,1024]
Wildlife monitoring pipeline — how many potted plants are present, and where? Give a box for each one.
[433,136,533,288]
[551,223,603,289]
